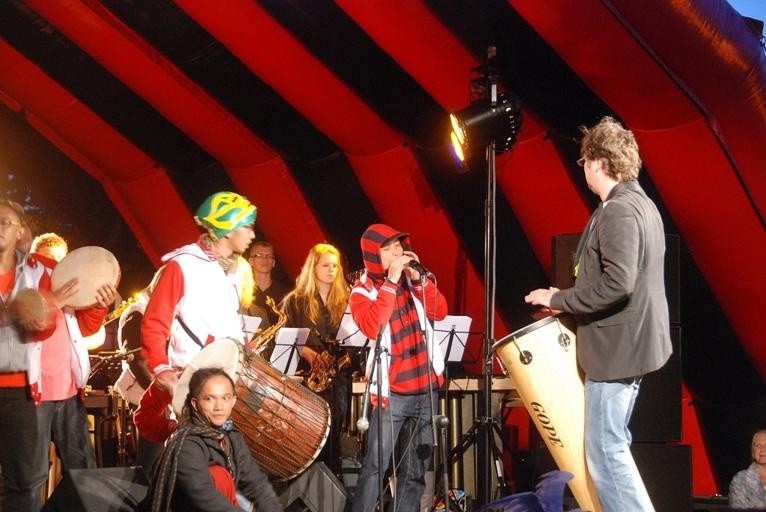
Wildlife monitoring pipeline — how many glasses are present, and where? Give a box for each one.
[244,225,255,230]
[253,252,274,260]
[577,157,585,167]
[0,220,21,227]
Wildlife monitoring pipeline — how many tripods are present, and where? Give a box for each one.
[436,145,531,499]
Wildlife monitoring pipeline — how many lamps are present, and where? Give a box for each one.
[450,94,518,145]
[447,127,523,163]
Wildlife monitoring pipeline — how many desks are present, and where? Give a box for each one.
[353,379,519,500]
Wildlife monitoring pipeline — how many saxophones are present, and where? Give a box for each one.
[304,351,351,391]
[248,297,287,353]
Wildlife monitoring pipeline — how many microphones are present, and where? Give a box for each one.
[356,384,370,437]
[409,259,434,279]
[99,351,135,363]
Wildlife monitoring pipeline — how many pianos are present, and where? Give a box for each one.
[353,376,514,394]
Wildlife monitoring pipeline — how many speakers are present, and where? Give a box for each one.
[551,233,680,325]
[626,327,682,442]
[629,443,693,512]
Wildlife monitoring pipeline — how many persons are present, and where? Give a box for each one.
[728,429,766,512]
[1,193,447,512]
[525,115,675,512]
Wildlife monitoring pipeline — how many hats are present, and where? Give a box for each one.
[194,191,256,241]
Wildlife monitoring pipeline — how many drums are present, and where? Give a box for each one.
[50,246,121,310]
[14,288,57,331]
[492,312,601,511]
[171,338,332,482]
